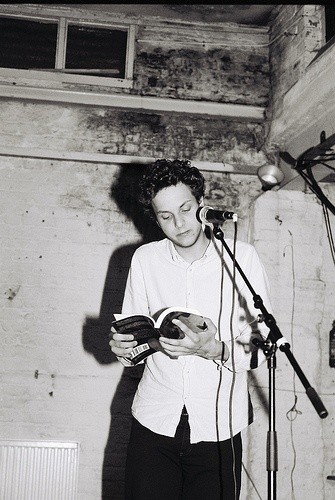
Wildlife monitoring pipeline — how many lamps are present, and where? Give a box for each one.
[257,165,284,192]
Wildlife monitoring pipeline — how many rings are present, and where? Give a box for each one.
[109,333,115,340]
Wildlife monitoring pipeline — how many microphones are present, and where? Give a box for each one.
[199,205,238,225]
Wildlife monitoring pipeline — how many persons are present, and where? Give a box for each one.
[108,158,273,500]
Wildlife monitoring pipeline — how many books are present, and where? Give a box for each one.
[112,306,210,363]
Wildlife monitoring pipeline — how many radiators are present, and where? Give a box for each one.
[0,439,80,500]
